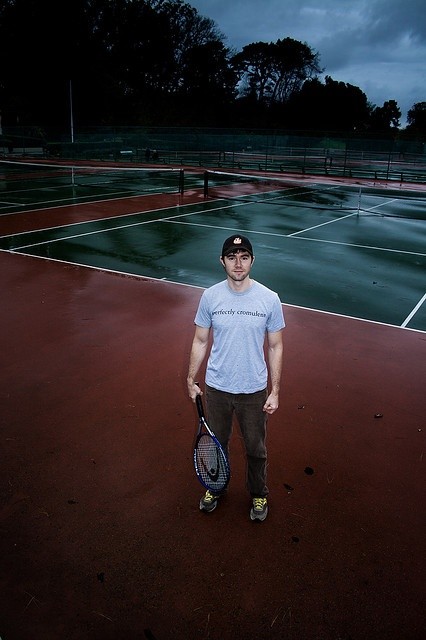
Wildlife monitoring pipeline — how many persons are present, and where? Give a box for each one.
[186,234,286,523]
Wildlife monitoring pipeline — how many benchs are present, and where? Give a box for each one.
[375,171,404,181]
[143,157,167,163]
[238,162,260,170]
[325,167,351,177]
[279,164,306,173]
[180,158,201,167]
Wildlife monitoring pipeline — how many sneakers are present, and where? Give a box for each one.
[250,493,268,522]
[199,489,222,513]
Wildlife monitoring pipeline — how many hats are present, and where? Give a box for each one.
[222,234,253,257]
